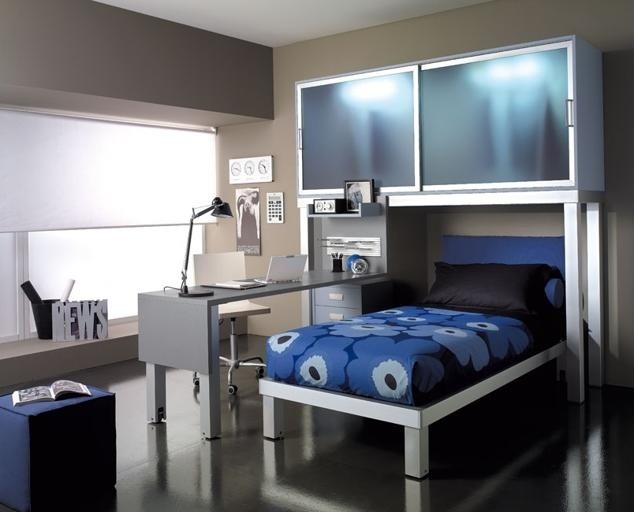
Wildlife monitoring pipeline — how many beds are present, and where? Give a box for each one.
[256,235,565,480]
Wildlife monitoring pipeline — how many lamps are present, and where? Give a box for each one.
[176,197,234,297]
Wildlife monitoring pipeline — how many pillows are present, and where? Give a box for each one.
[424,262,546,321]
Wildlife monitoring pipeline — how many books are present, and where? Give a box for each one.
[199,280,267,290]
[10,378,92,406]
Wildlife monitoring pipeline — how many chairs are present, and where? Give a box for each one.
[191,252,263,394]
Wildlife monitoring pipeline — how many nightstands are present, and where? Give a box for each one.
[313,278,390,323]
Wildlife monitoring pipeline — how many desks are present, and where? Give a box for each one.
[136,268,390,439]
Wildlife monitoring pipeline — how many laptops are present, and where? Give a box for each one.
[254,255,307,284]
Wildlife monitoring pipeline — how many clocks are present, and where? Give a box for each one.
[351,258,368,274]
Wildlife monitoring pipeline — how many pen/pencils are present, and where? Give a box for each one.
[331,253,343,259]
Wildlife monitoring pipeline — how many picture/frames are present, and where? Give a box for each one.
[345,178,374,212]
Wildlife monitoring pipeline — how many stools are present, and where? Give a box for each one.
[0,384,117,511]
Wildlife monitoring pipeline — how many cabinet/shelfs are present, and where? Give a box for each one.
[293,34,604,402]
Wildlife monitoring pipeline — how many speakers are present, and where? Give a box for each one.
[313,199,347,214]
